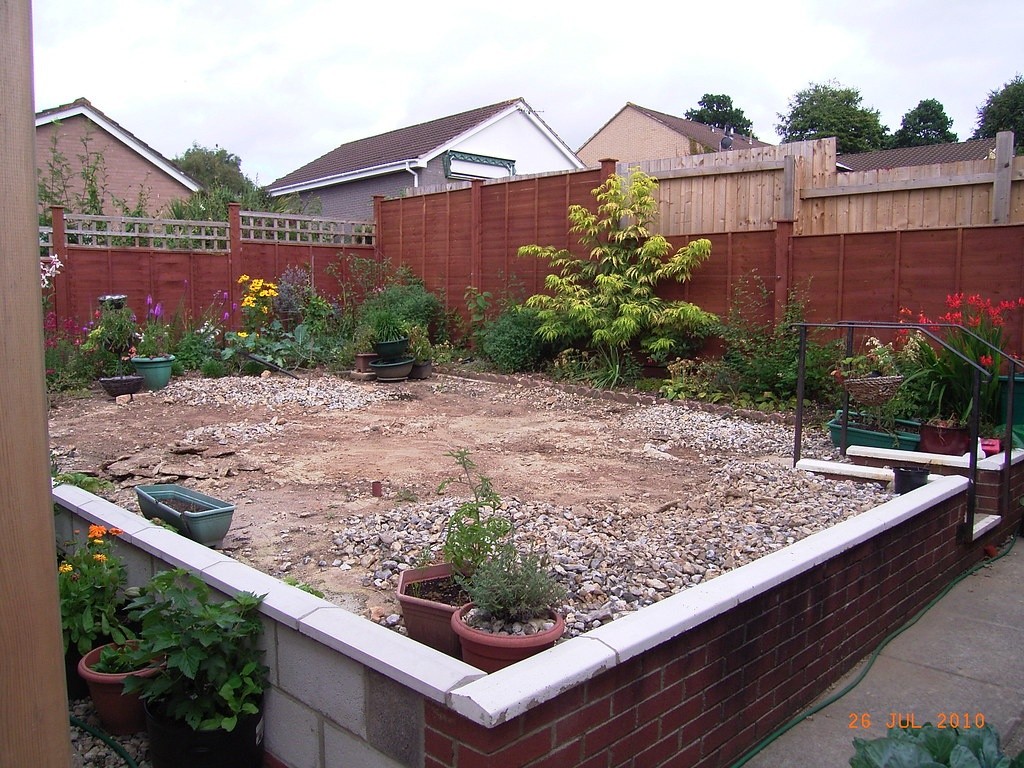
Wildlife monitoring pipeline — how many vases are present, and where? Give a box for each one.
[993,373,1024,426]
[920,419,970,457]
[134,484,236,546]
[66,612,134,701]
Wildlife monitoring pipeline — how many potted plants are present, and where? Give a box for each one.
[395,446,565,672]
[91,297,146,397]
[829,348,905,406]
[826,408,922,451]
[130,320,176,390]
[352,289,434,381]
[77,569,271,768]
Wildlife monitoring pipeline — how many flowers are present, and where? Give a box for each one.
[58,525,138,656]
[865,291,1024,438]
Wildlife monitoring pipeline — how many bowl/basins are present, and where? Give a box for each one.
[99,295,127,310]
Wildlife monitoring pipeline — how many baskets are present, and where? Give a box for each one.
[99,354,144,396]
[844,324,902,406]
